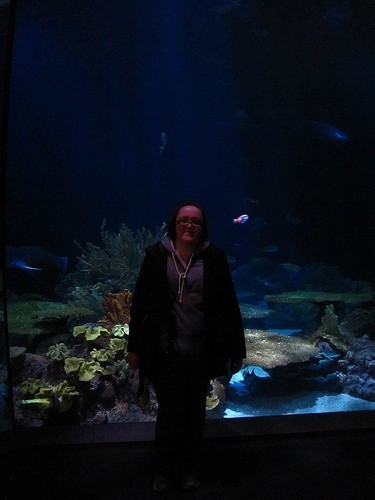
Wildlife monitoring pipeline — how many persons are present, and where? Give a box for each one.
[125,201,246,491]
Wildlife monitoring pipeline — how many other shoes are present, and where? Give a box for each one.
[181,472,200,489]
[153,474,170,490]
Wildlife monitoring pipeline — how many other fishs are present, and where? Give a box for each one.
[309,120,350,144]
[11,259,43,273]
[233,214,250,227]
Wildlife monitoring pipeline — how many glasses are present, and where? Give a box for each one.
[175,217,202,225]
